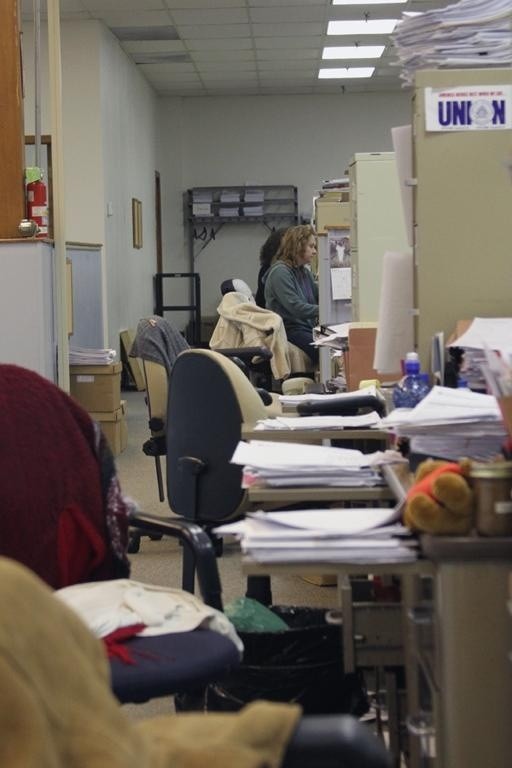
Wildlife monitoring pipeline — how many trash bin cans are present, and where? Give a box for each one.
[205,604,371,720]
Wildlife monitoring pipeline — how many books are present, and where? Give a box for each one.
[190,189,265,216]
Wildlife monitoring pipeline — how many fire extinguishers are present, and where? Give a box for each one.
[25,167,49,237]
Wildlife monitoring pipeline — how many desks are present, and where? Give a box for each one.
[239,385,508,767]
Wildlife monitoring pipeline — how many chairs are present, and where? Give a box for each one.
[162,350,338,608]
[223,280,275,384]
[1,364,244,717]
[133,316,274,504]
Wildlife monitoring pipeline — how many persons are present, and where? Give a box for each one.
[254,227,287,309]
[262,224,319,365]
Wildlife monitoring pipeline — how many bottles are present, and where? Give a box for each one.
[393,351,434,409]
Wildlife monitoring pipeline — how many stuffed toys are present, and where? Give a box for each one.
[397,457,475,537]
